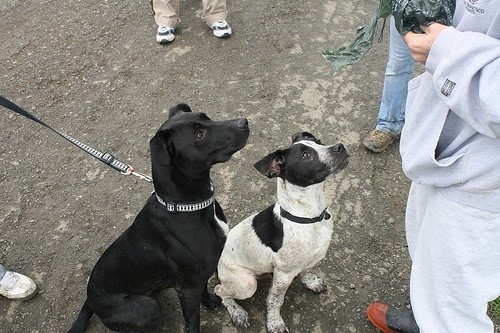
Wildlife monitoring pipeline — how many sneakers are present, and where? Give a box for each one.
[0,271,38,301]
[206,20,232,37]
[156,25,177,43]
[363,128,399,152]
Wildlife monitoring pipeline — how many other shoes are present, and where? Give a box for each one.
[367,303,401,333]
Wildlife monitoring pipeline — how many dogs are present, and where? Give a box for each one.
[68,102,251,333]
[213,132,350,333]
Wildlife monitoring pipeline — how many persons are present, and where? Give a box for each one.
[362,0,500,333]
[152,0,231,43]
[0,264,38,301]
